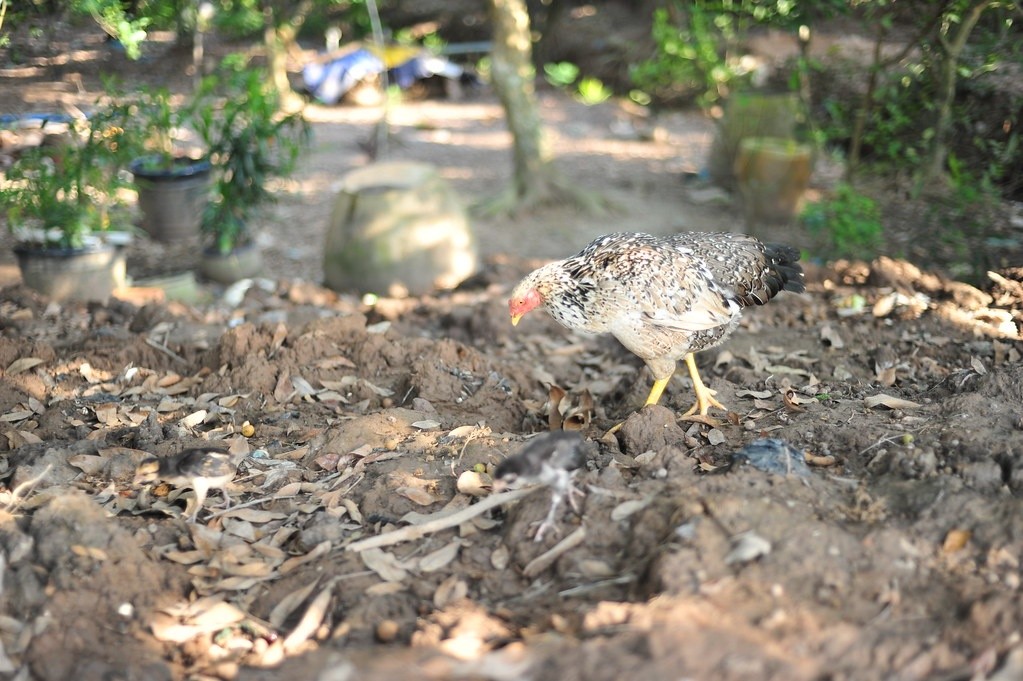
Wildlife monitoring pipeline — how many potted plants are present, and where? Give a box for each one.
[0,52,316,301]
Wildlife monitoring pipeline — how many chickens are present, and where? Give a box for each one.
[510,230,809,444]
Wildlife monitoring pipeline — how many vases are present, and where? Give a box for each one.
[705,85,813,220]
